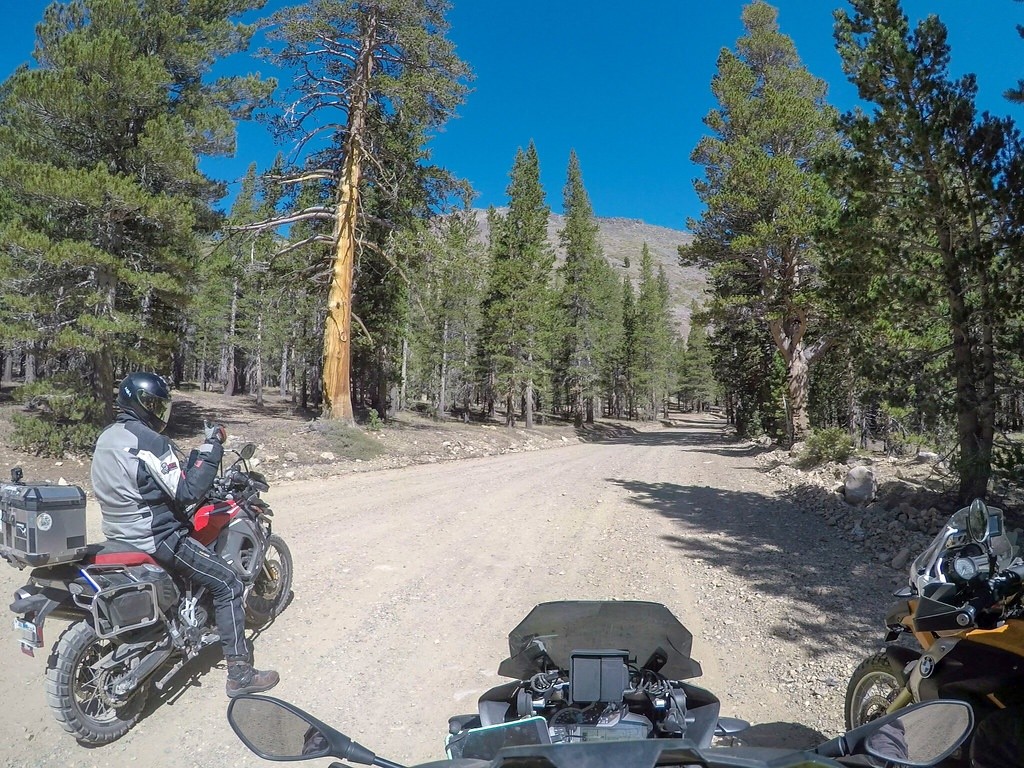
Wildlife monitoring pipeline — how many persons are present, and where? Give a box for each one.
[91,372,279,699]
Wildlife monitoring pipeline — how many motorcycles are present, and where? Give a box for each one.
[0,442,294,746]
[846,494,1024,768]
[226,599,974,768]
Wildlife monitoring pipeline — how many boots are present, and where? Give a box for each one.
[225,653,279,698]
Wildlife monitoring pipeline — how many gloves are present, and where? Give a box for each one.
[203,418,227,444]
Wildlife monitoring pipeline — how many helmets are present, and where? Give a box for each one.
[115,372,173,434]
